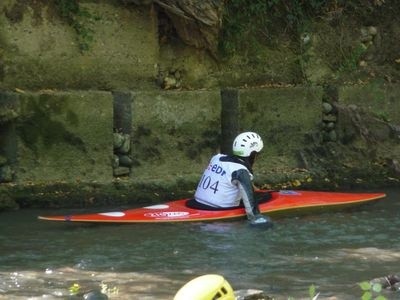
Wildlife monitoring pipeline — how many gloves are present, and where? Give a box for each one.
[249,215,273,230]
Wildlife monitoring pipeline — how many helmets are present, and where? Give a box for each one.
[232,132,264,156]
[173,274,234,300]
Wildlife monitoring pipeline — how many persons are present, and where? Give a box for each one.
[193,131,274,229]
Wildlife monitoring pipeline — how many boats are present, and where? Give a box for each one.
[37,190,388,224]
